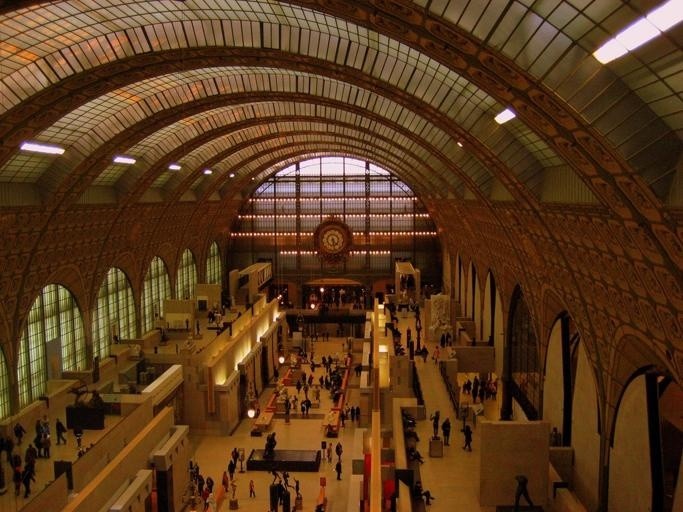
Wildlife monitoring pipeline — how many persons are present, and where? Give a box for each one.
[1,390,103,499]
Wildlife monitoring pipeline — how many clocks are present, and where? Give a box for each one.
[312,217,353,262]
